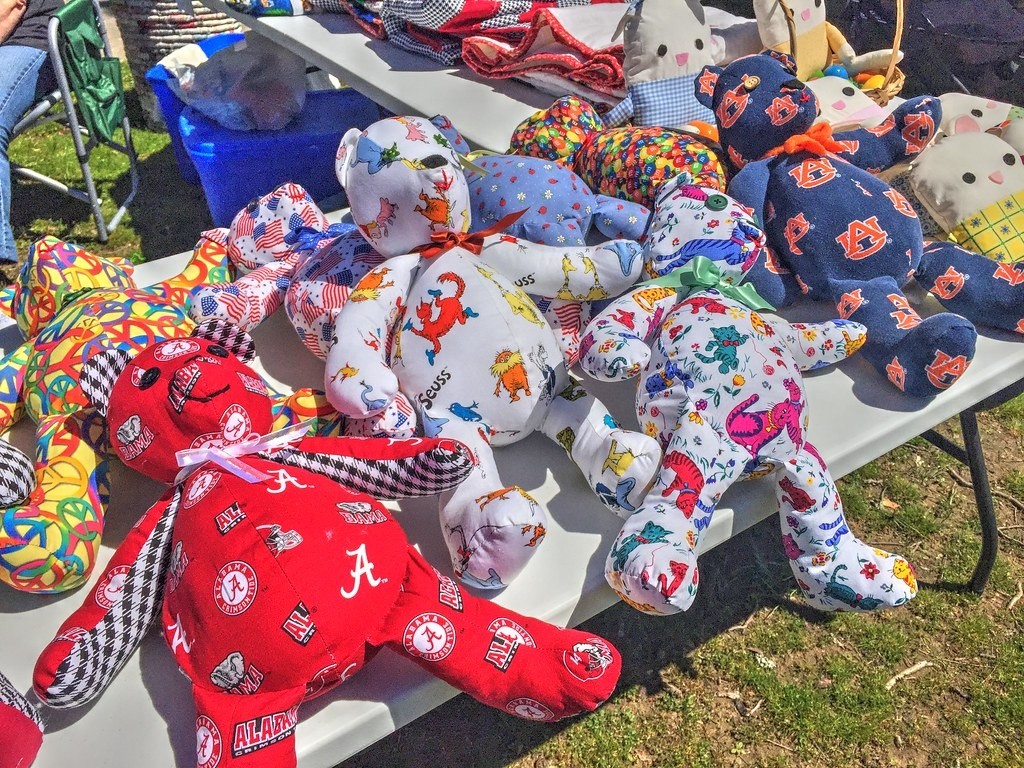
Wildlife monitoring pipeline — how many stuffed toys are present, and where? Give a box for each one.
[0,0,1024,767]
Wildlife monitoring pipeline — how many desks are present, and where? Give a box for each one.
[199,0,553,153]
[1,96,1024,768]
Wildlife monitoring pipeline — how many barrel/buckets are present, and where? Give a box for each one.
[145,33,392,235]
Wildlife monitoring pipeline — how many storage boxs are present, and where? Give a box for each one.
[146,32,245,183]
[179,88,381,229]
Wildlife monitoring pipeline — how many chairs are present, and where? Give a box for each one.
[9,1,139,245]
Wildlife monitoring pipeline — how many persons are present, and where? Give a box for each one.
[0,0,66,282]
